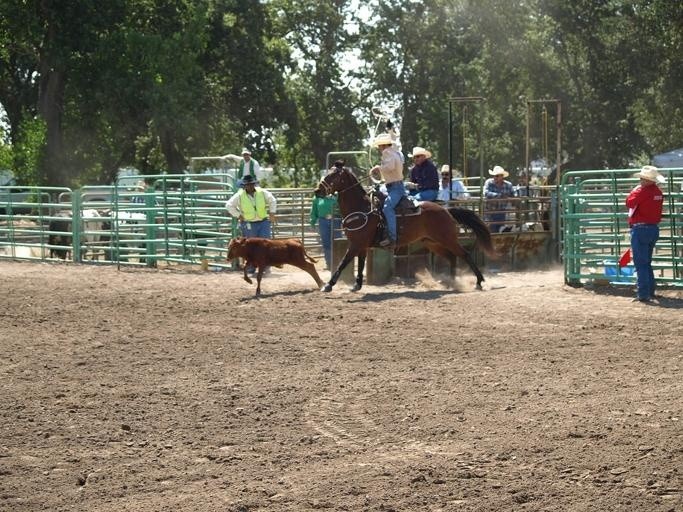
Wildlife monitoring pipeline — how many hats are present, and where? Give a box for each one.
[237,175,257,186]
[488,165,509,178]
[374,133,395,146]
[516,170,532,177]
[408,146,432,159]
[633,165,666,184]
[441,164,457,174]
[241,148,251,155]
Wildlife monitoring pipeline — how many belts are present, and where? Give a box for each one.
[386,180,402,187]
[242,217,268,223]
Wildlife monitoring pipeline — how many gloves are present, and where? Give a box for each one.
[407,182,419,190]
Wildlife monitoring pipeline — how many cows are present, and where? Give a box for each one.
[224,237,326,297]
[45,209,148,264]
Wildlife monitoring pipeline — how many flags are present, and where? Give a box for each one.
[618,249,631,268]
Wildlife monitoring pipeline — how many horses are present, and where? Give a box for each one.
[314,159,495,295]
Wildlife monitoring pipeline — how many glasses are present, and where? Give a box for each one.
[442,173,448,176]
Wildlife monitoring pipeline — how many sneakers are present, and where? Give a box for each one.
[379,238,396,246]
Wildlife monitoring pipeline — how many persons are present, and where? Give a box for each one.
[482,166,513,233]
[405,146,439,201]
[371,116,403,249]
[222,149,262,187]
[309,176,343,270]
[623,165,666,303]
[513,170,534,216]
[129,181,158,264]
[437,164,469,206]
[224,175,277,278]
